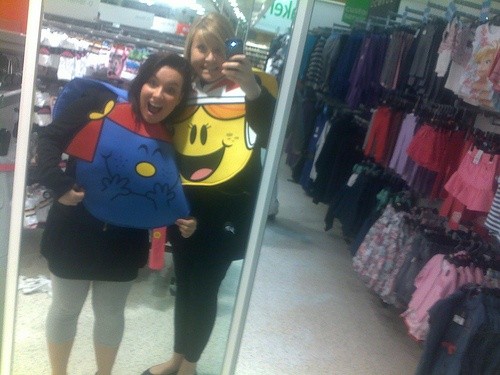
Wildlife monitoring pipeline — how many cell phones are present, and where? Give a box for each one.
[225,39,244,63]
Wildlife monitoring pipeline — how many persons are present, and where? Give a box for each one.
[138,11,277,375]
[472,47,494,107]
[34,51,198,375]
[265,81,307,220]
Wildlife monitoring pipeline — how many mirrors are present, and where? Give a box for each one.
[1,0,317,375]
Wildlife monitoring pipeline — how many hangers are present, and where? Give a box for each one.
[419,103,500,268]
